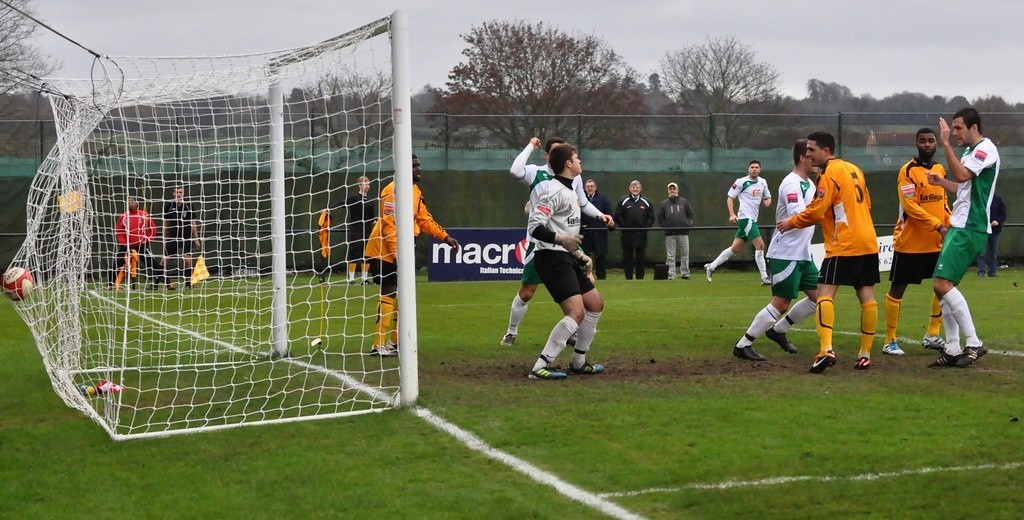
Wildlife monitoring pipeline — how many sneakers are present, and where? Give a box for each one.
[760,276,772,285]
[528,365,567,380]
[370,339,397,357]
[882,341,905,356]
[704,263,712,283]
[566,331,577,348]
[569,361,604,374]
[927,340,987,368]
[349,280,376,285]
[809,350,837,374]
[854,356,871,370]
[733,341,767,361]
[922,334,946,352]
[501,333,517,346]
[765,325,798,353]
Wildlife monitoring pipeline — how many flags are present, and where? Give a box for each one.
[190,255,210,287]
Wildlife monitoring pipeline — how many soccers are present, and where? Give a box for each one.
[1,268,34,301]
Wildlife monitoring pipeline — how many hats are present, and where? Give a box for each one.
[668,182,678,189]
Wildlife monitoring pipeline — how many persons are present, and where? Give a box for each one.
[658,182,694,280]
[614,180,655,280]
[882,108,1007,368]
[104,186,201,290]
[499,136,615,378]
[579,179,616,279]
[319,176,375,285]
[364,153,458,355]
[703,160,771,286]
[732,131,881,375]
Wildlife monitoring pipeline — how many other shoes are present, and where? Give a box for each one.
[978,273,999,278]
[186,285,189,290]
[97,287,112,291]
[146,285,158,290]
[167,283,177,290]
